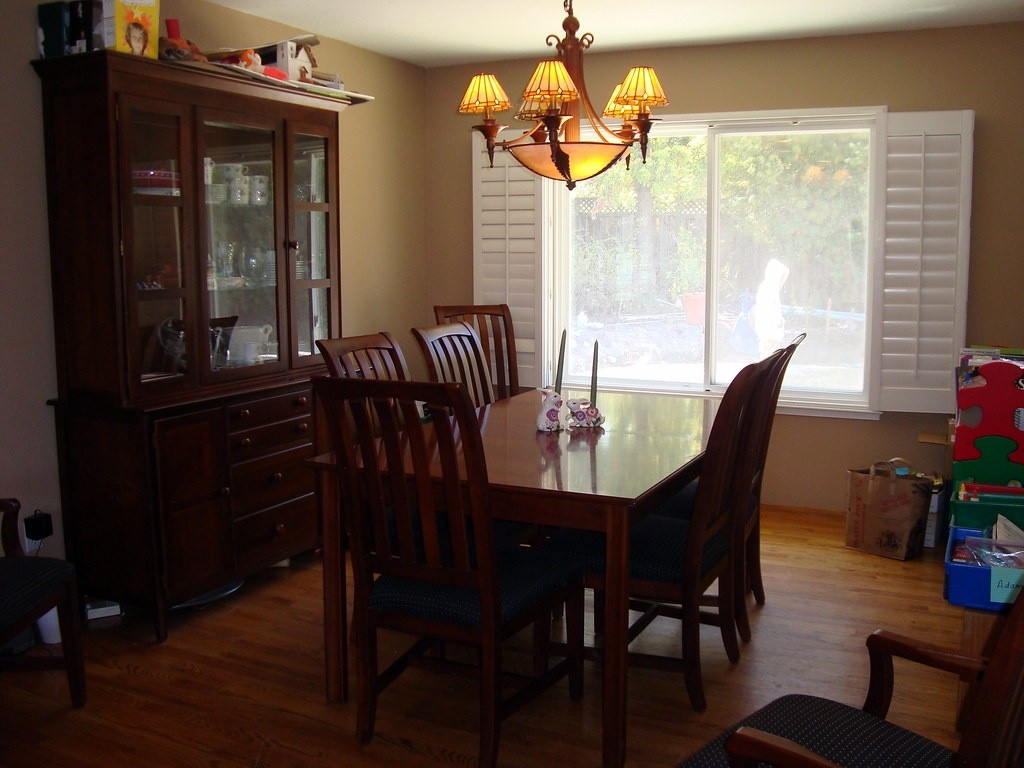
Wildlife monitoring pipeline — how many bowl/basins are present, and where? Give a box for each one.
[227,175,270,206]
[132,169,180,188]
[219,324,271,351]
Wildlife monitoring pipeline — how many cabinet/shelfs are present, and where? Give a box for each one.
[30,48,348,645]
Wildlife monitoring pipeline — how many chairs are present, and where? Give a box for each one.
[434,303,520,401]
[652,334,810,643]
[675,585,1023,767]
[315,328,542,583]
[412,321,567,546]
[2,497,91,709]
[556,345,791,711]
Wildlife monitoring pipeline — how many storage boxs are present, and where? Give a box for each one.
[949,491,1024,539]
[943,527,1024,613]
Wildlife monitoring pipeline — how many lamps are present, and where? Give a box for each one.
[456,0,670,193]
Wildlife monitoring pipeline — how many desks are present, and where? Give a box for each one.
[300,384,734,767]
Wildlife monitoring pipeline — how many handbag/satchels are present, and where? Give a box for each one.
[843,456,935,563]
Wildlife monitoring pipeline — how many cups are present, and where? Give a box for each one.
[219,239,235,276]
[203,157,216,184]
[225,163,249,176]
[240,241,266,287]
[244,342,257,362]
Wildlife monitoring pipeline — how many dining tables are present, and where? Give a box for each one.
[309,376,585,768]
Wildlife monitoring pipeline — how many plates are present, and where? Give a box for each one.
[266,260,305,282]
[203,183,228,205]
[216,276,244,289]
